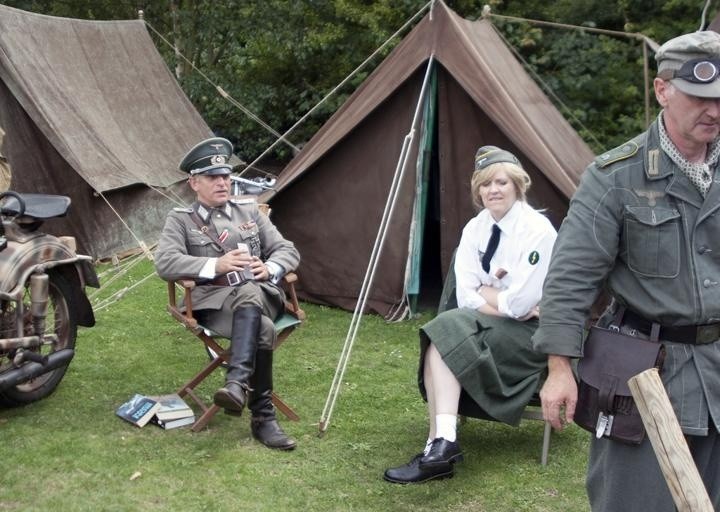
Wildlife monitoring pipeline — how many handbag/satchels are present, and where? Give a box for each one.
[573,324,667,448]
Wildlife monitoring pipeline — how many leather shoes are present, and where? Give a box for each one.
[383,437,464,484]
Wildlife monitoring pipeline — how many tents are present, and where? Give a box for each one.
[260,0,596,322]
[0,5,246,262]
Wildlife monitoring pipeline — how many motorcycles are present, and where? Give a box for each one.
[0,154,100,403]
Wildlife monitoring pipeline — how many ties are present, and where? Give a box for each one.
[482,223,501,273]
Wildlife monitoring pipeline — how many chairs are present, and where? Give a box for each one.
[167,202,305,432]
[435,214,570,463]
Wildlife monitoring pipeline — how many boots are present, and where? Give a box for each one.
[214,306,262,418]
[247,348,297,450]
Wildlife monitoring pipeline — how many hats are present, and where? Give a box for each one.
[471,145,523,172]
[654,30,720,98]
[177,137,234,175]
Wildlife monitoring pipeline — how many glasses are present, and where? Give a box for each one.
[656,58,719,83]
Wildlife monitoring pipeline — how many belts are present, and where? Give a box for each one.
[622,310,720,345]
[205,269,246,287]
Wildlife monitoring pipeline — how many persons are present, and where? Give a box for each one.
[155,137,301,450]
[383,145,557,484]
[530,29,720,512]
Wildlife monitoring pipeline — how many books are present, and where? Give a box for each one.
[114,393,195,430]
